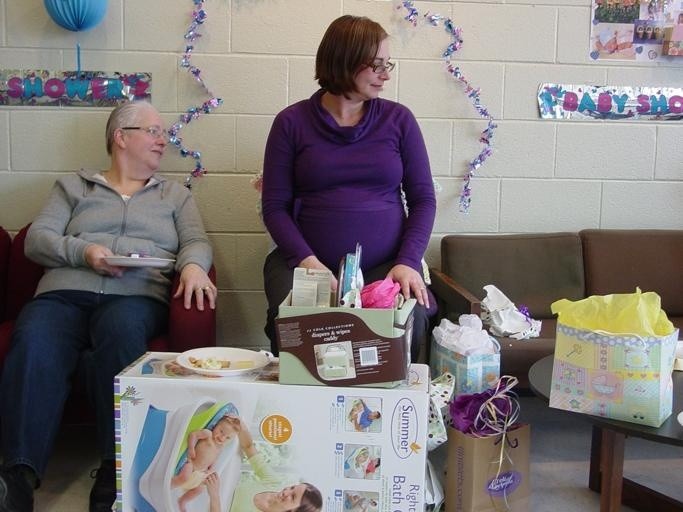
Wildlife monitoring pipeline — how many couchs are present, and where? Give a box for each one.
[428,229,682,389]
[0,222,215,437]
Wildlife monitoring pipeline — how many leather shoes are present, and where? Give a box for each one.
[88,462,118,511]
[2,470,32,511]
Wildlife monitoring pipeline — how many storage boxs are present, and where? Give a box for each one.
[114,352,428,511]
[274,290,417,386]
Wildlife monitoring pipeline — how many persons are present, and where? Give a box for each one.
[0,100,220,512]
[260,16,437,378]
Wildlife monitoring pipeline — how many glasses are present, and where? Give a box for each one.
[366,60,397,75]
[122,125,170,140]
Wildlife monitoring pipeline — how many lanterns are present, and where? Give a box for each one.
[44,0,108,77]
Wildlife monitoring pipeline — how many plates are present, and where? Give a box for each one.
[177,346,270,378]
[101,256,177,269]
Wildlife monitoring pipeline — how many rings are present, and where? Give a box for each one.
[203,286,210,290]
[195,288,204,291]
